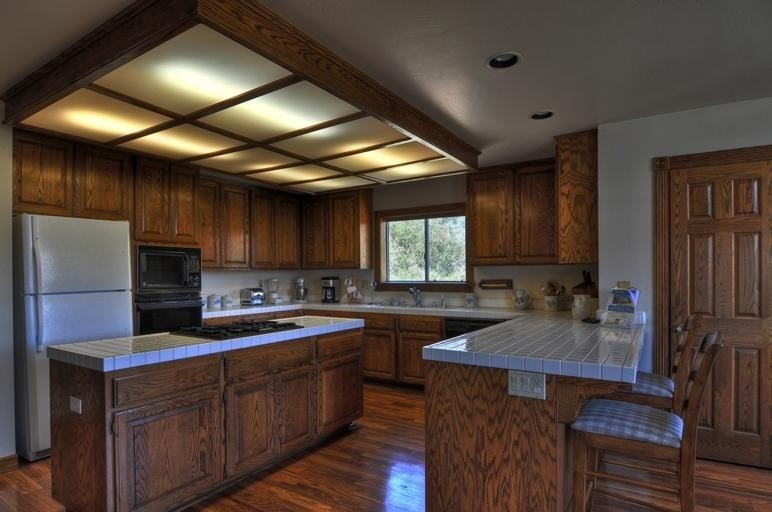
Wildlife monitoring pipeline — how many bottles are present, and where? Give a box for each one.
[201,293,233,310]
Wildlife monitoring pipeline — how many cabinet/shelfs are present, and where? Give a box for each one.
[226,333,315,493]
[196,173,249,270]
[466,159,552,267]
[304,188,372,269]
[424,346,633,512]
[12,128,134,220]
[553,129,600,264]
[351,309,444,386]
[316,319,364,453]
[250,179,305,270]
[50,357,225,512]
[134,152,196,248]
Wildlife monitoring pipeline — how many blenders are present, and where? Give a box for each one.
[268,278,283,304]
[291,277,308,302]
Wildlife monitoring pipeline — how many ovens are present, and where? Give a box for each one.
[135,301,206,335]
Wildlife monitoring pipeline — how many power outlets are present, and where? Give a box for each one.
[69,396,82,415]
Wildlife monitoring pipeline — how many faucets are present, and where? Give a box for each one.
[409,288,423,307]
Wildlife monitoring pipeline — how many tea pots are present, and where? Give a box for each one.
[510,288,532,310]
[571,294,591,320]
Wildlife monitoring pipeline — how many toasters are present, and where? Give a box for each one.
[240,287,265,306]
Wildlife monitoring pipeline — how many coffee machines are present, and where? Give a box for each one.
[320,277,340,303]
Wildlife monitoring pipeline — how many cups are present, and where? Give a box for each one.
[545,296,558,312]
[464,293,475,308]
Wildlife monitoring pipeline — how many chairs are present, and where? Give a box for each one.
[599,310,705,413]
[570,331,724,512]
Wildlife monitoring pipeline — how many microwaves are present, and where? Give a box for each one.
[135,244,202,294]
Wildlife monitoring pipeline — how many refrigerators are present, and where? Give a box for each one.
[14,213,134,463]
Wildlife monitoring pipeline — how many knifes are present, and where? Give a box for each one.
[582,270,592,286]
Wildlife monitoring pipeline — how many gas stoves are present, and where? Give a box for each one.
[170,320,304,341]
